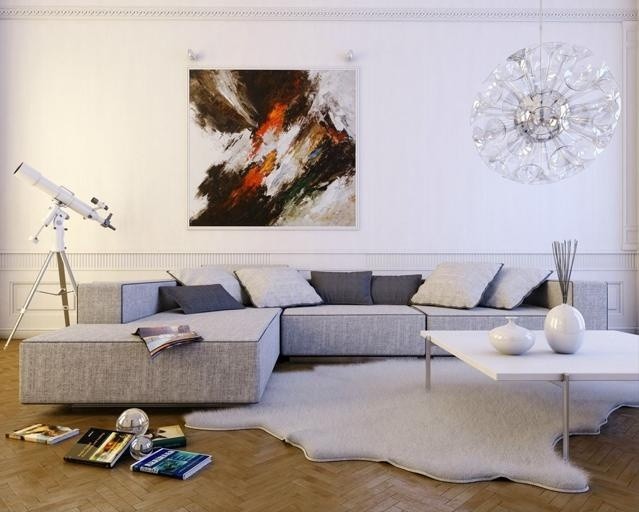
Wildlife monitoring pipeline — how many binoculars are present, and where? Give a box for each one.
[14,162,116,231]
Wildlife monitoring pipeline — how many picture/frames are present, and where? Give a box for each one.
[183,64,361,232]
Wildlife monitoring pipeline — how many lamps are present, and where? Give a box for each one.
[465,0,624,189]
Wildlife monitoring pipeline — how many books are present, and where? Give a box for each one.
[131,325,204,359]
[130,447,212,481]
[63,427,135,469]
[5,422,80,446]
[145,424,187,447]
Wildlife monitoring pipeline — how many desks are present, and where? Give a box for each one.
[416,326,639,463]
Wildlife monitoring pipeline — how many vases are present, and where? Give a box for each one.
[542,303,587,354]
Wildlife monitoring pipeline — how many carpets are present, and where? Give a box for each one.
[179,349,639,496]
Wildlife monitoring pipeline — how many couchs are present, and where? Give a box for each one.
[16,277,610,412]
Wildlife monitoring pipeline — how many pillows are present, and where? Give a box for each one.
[369,274,423,306]
[477,266,554,309]
[165,267,251,307]
[232,266,325,308]
[156,283,245,317]
[406,261,504,311]
[309,270,374,306]
